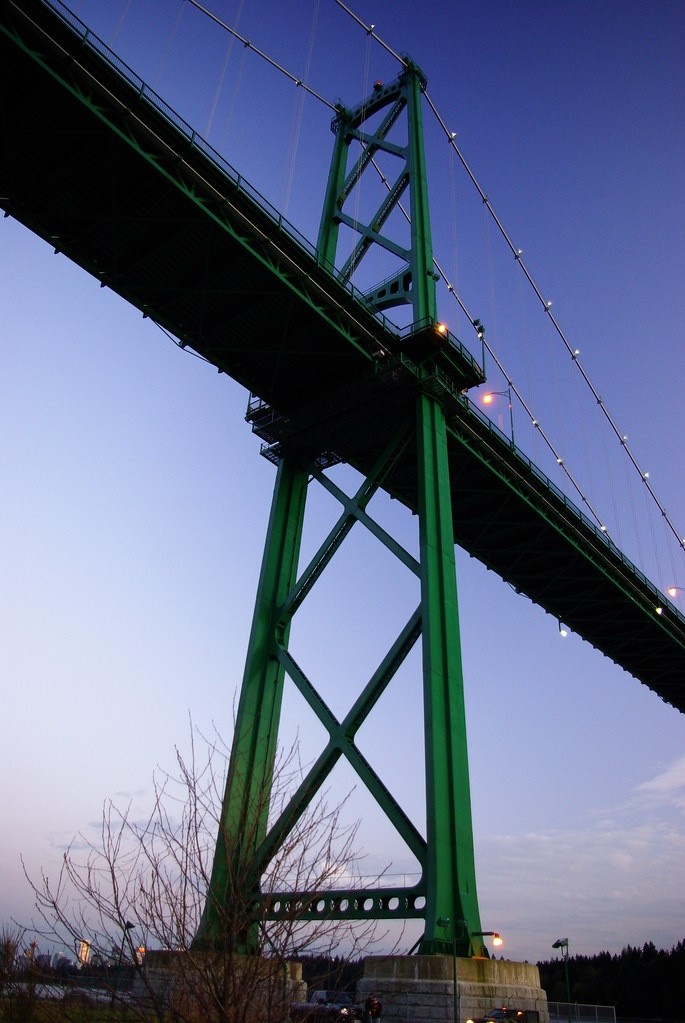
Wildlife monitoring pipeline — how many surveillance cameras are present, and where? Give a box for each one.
[552,938,568,948]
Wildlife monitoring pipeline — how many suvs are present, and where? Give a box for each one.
[63,988,112,1004]
[466,1008,540,1023]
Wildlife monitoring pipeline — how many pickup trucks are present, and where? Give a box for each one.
[290,991,366,1023]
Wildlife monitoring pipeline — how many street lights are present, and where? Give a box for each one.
[435,917,468,1023]
[474,318,485,380]
[483,389,514,452]
[552,938,574,1023]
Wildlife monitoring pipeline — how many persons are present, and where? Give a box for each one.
[351,992,383,1023]
